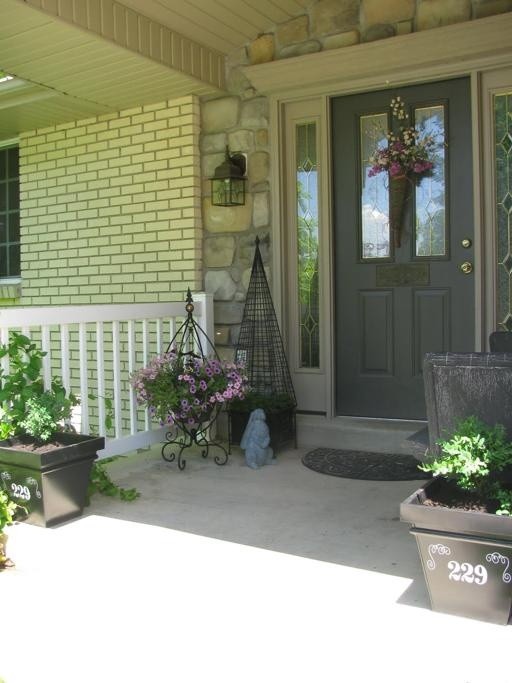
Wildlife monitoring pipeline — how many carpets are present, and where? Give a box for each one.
[302,446,434,480]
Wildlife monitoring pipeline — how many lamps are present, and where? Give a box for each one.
[209,142,248,207]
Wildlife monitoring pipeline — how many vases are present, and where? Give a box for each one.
[176,402,216,422]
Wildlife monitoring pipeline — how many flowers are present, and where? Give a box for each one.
[128,350,251,429]
[364,96,449,186]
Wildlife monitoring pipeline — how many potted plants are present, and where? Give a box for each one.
[398,414,512,625]
[228,389,296,447]
[0,331,143,528]
